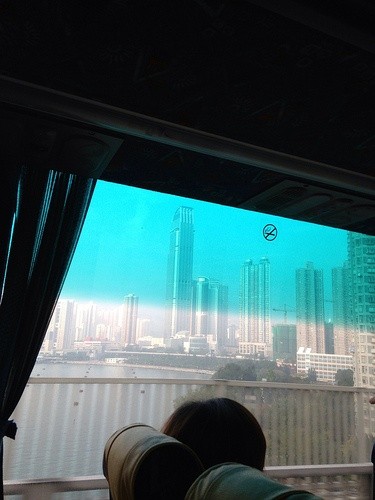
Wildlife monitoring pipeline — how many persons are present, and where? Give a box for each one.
[161,394,268,473]
